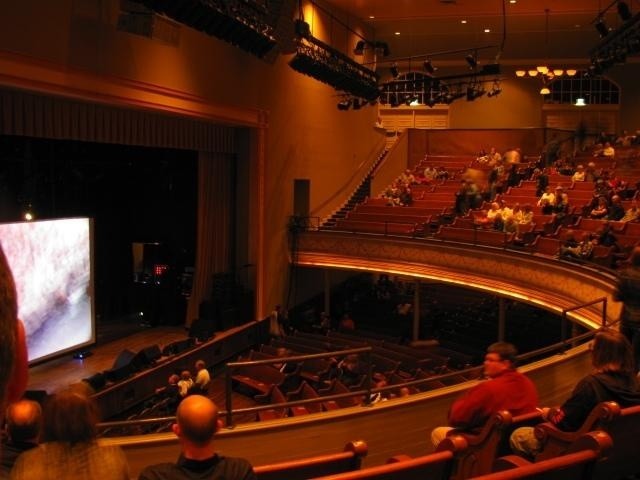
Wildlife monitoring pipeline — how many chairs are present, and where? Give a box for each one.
[99,150,640,480]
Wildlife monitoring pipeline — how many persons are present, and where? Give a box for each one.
[176,370,193,397]
[0,398,40,474]
[427,351,446,373]
[608,265,640,373]
[320,312,329,327]
[142,374,179,409]
[138,392,257,480]
[273,340,411,408]
[193,360,212,388]
[341,313,355,330]
[8,387,135,479]
[446,350,463,370]
[429,343,540,452]
[380,130,640,266]
[505,327,640,455]
[0,243,30,466]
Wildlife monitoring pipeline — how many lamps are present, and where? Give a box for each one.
[139,0,501,111]
[513,0,640,100]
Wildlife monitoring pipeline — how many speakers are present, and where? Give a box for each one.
[86,271,241,394]
[24,391,46,402]
